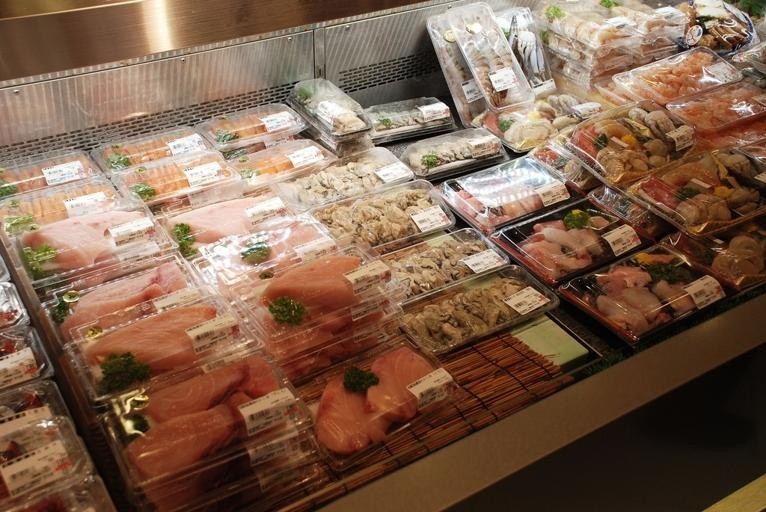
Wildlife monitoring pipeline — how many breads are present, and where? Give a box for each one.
[537,0,751,88]
